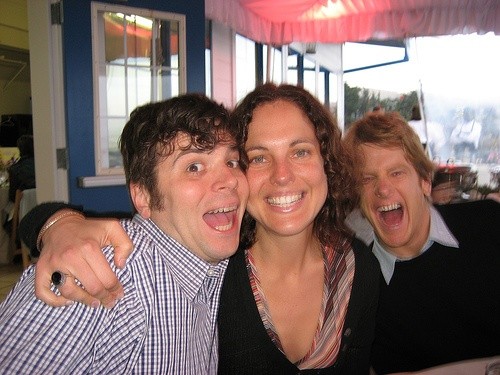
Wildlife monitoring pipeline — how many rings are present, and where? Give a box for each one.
[51,269,74,288]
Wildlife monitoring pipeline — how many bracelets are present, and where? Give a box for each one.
[36,209,83,252]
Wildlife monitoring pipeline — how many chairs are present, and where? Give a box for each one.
[9,188,36,270]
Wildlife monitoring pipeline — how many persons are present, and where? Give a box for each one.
[3,136,36,264]
[0,84,500,375]
